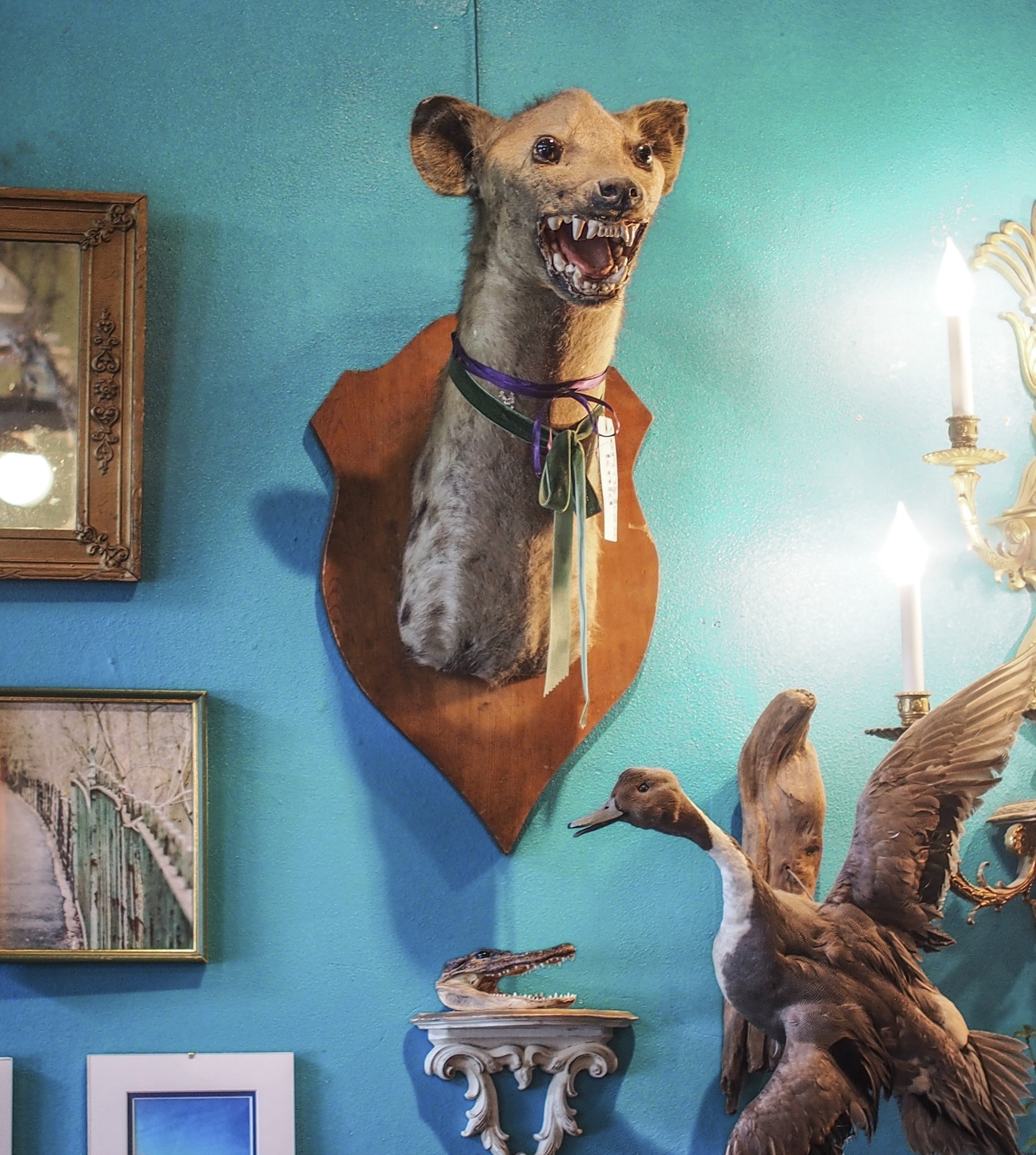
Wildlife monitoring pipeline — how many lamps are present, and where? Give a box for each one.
[863,200,1036,926]
[0,434,53,507]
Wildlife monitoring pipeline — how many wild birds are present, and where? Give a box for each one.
[566,650,1036,1155]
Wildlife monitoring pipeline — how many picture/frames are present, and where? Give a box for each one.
[0,685,210,964]
[86,1053,296,1154]
[1,187,145,582]
